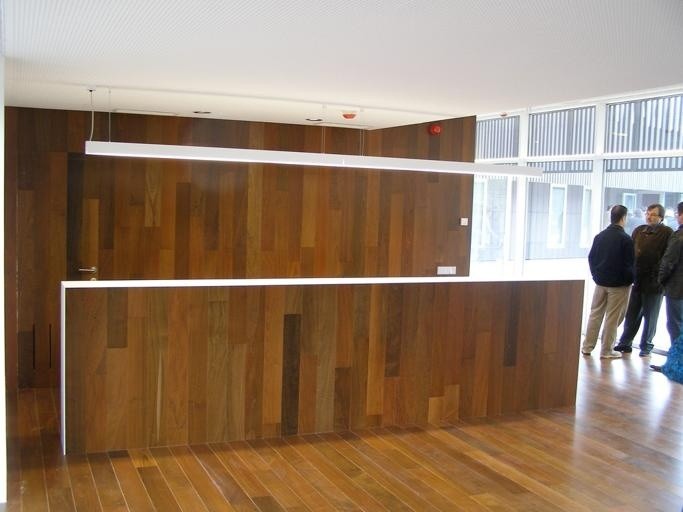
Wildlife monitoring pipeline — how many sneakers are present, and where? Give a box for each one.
[582,349,590,355]
[601,350,622,358]
[614,346,632,352]
[639,351,649,356]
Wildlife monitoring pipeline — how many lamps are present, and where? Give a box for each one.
[82,88,546,182]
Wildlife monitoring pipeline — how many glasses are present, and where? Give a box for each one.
[645,213,660,217]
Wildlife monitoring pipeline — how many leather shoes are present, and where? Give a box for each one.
[650,365,661,371]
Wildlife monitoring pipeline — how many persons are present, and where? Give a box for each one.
[614,203,674,357]
[581,205,634,358]
[649,201,683,371]
